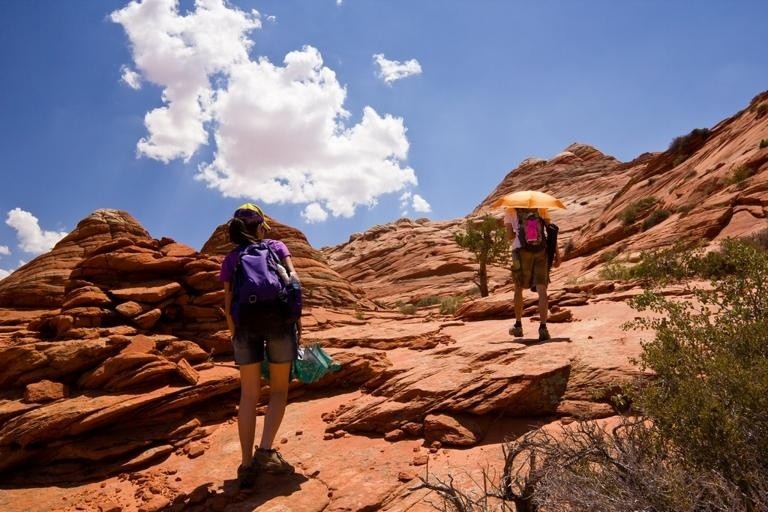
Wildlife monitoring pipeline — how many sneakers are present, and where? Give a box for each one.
[236,462,282,493]
[538,328,551,341]
[508,326,524,337]
[252,447,296,475]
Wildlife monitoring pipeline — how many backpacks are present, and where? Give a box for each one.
[519,213,545,253]
[229,243,304,334]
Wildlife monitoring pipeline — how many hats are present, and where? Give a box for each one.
[233,202,272,232]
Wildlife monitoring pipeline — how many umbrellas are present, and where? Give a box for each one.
[489,187,565,212]
[256,339,341,384]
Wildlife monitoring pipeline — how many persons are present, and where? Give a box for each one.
[502,208,561,342]
[218,201,304,496]
